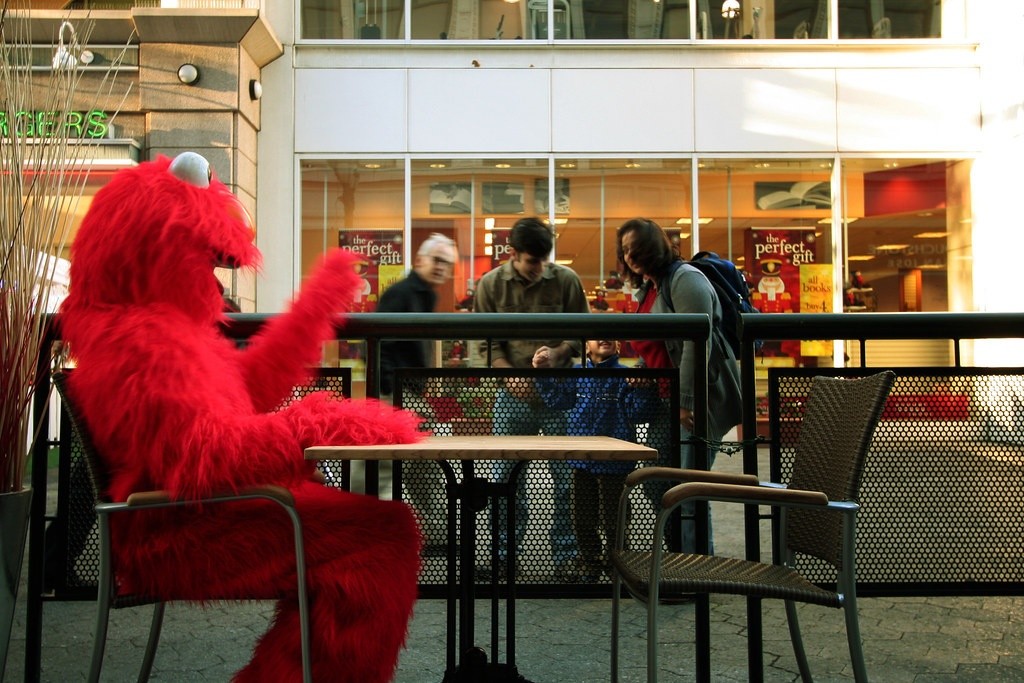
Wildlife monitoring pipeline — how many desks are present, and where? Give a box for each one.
[303,435,659,683]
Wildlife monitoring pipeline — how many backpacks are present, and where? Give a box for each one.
[662,250,764,359]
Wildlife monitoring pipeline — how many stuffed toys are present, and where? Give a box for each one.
[59,148,427,683]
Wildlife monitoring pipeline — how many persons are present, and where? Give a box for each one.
[26,299,241,602]
[534,339,661,582]
[615,217,743,556]
[343,233,463,557]
[457,216,591,582]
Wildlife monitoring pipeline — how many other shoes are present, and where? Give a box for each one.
[474,564,519,578]
[422,545,465,557]
[555,564,586,578]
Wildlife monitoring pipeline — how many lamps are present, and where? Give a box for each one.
[249,79,263,99]
[177,63,201,86]
[51,19,93,68]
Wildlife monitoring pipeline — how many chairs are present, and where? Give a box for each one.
[51,373,311,683]
[609,368,897,683]
